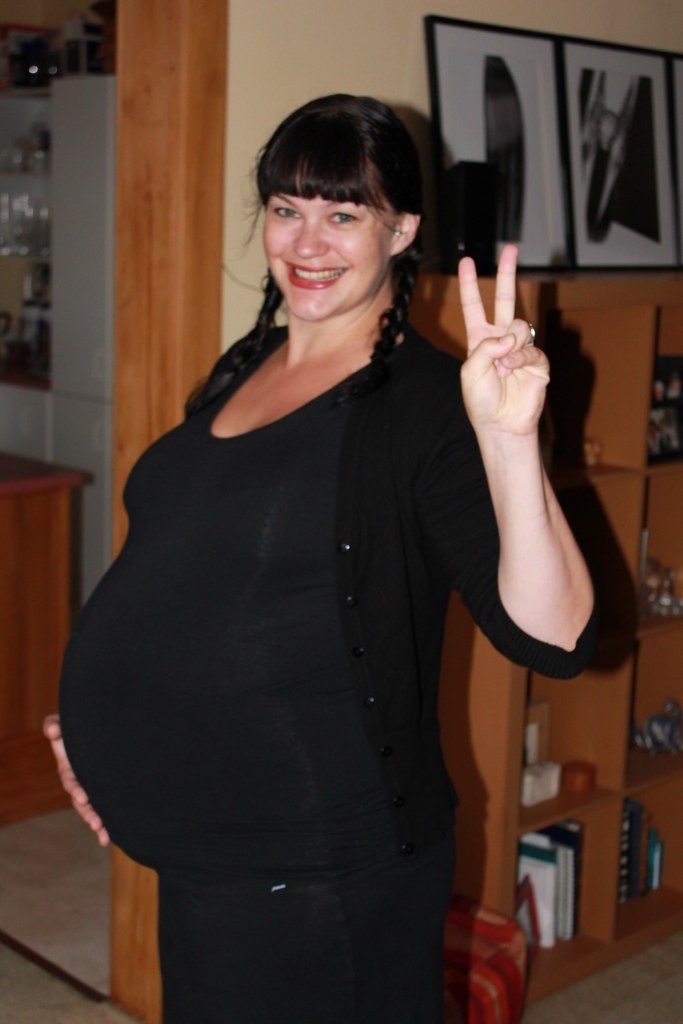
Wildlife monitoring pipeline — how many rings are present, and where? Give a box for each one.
[522,320,536,345]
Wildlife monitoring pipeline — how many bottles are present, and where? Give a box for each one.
[648,570,683,618]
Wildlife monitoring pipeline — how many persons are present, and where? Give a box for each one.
[45,96,605,1024]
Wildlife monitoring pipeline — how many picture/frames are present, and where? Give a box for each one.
[562,33,678,273]
[671,52,683,229]
[422,15,579,277]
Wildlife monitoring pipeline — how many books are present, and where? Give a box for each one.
[616,799,663,911]
[517,820,587,954]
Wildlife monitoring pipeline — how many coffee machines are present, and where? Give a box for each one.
[4,264,51,381]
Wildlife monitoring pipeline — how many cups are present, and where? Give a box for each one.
[0,146,49,175]
[0,192,50,257]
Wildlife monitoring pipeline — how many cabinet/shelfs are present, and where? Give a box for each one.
[403,273,681,1009]
